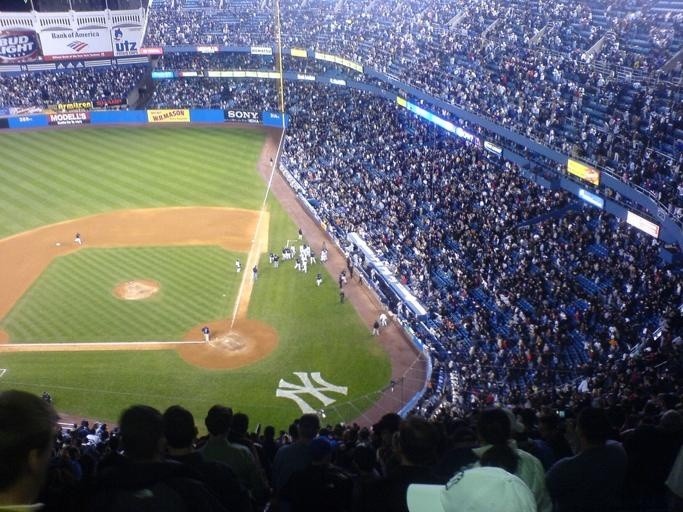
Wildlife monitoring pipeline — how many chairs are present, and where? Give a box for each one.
[282,0,683,291]
[427,158,683,422]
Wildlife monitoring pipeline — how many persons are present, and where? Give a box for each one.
[269,251,274,264]
[199,325,211,344]
[272,254,279,268]
[278,1,683,389]
[268,157,273,167]
[0,0,278,118]
[74,232,82,246]
[252,265,257,280]
[235,259,241,273]
[0,390,683,512]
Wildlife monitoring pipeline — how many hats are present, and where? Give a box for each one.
[405,465,537,512]
